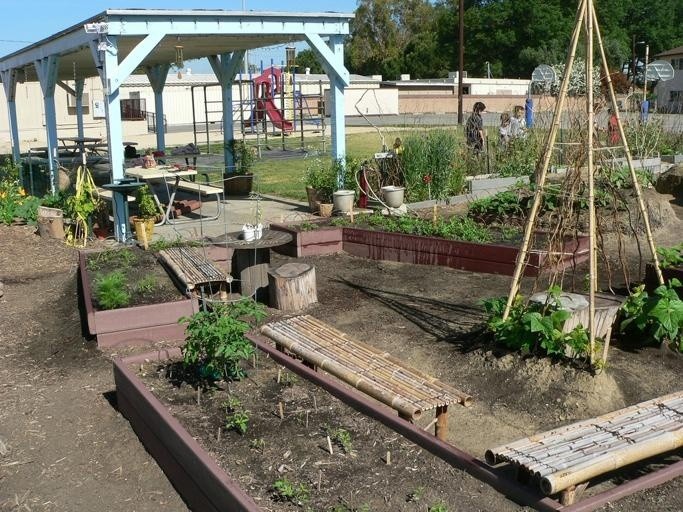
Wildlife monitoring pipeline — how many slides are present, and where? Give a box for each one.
[243,104,263,127]
[260,99,293,133]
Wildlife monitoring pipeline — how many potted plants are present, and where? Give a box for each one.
[132,185,158,242]
[305,158,343,217]
[645,242,683,301]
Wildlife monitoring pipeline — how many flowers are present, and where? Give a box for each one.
[223,139,259,173]
[301,159,326,187]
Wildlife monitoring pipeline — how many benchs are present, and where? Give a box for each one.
[30,142,138,159]
[73,182,136,223]
[159,246,234,317]
[260,315,474,442]
[157,180,225,218]
[485,390,683,506]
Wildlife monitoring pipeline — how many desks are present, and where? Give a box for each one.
[210,228,294,305]
[124,165,197,225]
[141,152,201,181]
[58,137,103,157]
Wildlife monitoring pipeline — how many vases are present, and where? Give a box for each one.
[381,185,407,208]
[307,185,322,209]
[224,170,254,195]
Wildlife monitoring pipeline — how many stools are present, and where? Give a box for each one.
[266,261,319,311]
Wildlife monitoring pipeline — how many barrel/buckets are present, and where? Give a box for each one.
[383,186,406,207]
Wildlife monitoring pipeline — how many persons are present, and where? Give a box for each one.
[587,104,606,141]
[462,101,487,162]
[497,112,511,156]
[606,108,620,144]
[511,106,529,139]
